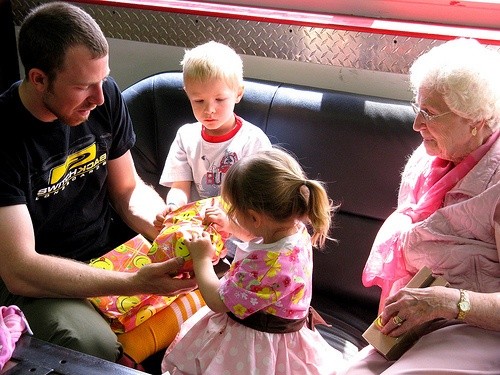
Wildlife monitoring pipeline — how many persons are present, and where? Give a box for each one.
[0,3,201,364]
[345,38,500,375]
[153,40,282,253]
[160,148,336,375]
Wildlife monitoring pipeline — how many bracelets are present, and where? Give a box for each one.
[167,202,182,209]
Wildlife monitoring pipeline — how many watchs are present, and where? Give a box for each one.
[456,288,470,322]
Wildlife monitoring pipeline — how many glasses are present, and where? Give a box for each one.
[410,97,451,122]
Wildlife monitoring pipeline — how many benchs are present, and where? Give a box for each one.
[120,71,423,331]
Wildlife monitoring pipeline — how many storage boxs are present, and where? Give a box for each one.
[362,266,451,361]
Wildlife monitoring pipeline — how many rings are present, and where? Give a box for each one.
[392,315,403,326]
